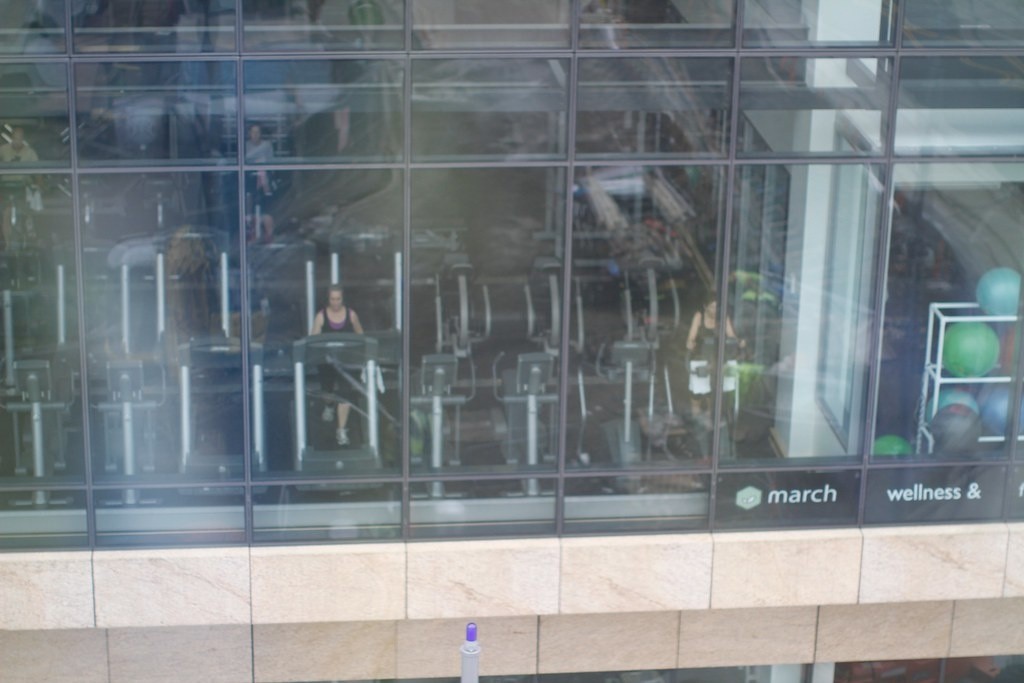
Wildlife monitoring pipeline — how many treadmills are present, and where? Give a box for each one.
[1,342,742,490]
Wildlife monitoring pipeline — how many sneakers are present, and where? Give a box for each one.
[322,405,335,423]
[334,428,349,445]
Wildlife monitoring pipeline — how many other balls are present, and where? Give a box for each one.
[925,387,977,421]
[1001,329,1016,374]
[975,267,1021,315]
[930,403,979,454]
[978,383,1024,437]
[873,434,912,455]
[942,322,999,377]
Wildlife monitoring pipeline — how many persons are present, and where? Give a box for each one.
[18,0,275,138]
[0,126,43,250]
[685,291,745,432]
[332,62,370,177]
[243,123,279,245]
[311,284,364,444]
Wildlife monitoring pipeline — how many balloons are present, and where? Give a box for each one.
[867,264,1022,458]
